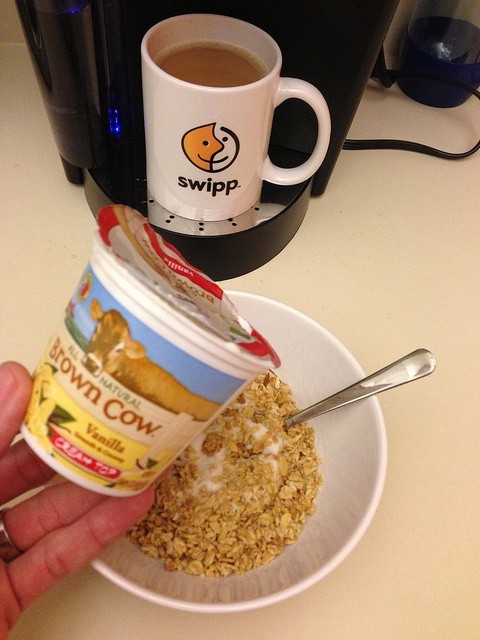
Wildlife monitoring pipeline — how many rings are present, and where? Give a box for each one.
[1,507,24,562]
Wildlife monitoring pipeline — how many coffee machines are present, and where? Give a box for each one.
[15,0,401,283]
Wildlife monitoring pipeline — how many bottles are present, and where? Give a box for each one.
[397,0,480,109]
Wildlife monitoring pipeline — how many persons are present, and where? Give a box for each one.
[1,361,156,640]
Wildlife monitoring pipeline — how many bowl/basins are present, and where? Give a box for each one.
[43,286,389,614]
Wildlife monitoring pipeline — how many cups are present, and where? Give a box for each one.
[140,12,333,223]
